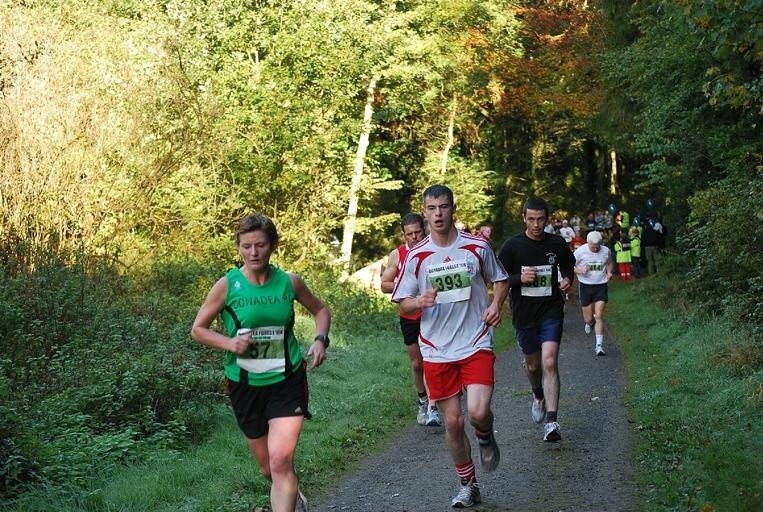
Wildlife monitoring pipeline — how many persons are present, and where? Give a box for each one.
[190,212,330,512]
[496,196,576,444]
[381,213,442,427]
[390,185,510,509]
[544,207,668,357]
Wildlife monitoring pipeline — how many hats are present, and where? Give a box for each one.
[562,220,568,226]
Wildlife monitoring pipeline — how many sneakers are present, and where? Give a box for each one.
[416,399,444,429]
[474,432,501,476]
[583,322,596,336]
[450,473,482,510]
[543,421,562,443]
[594,342,607,360]
[530,396,547,423]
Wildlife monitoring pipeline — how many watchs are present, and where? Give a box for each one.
[315,335,330,349]
